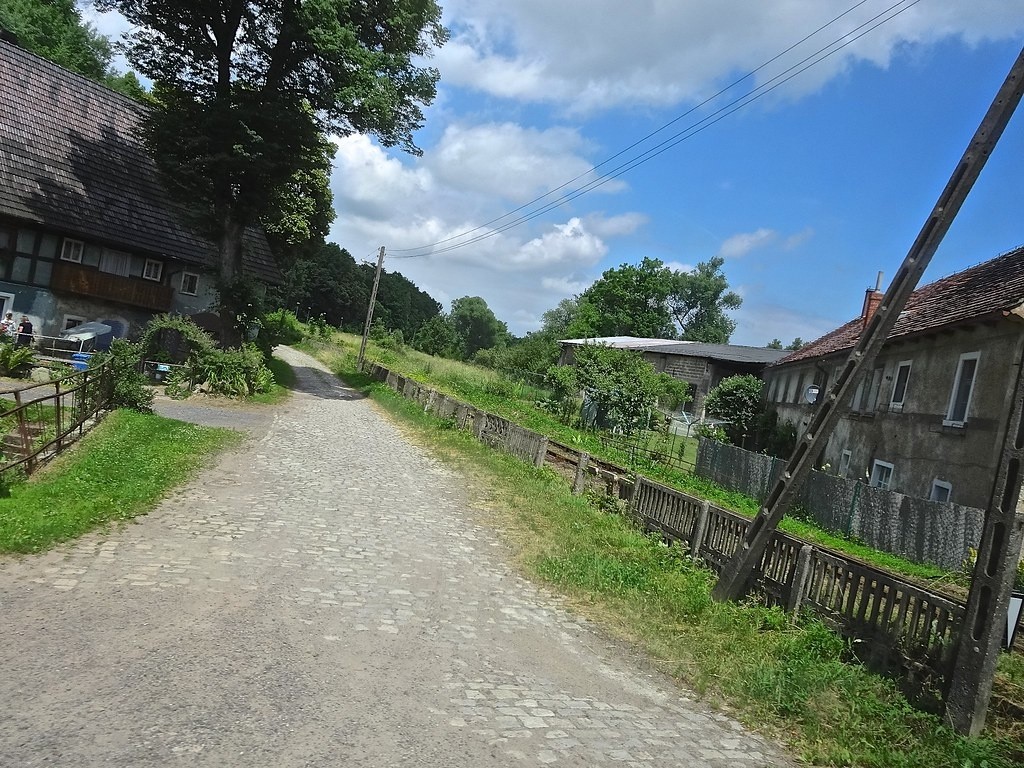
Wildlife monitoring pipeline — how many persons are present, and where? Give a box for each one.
[17,316,34,348]
[0,313,16,343]
